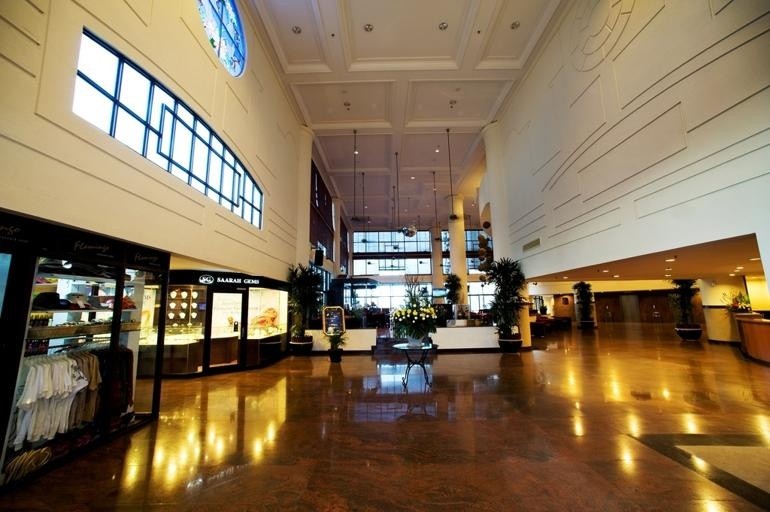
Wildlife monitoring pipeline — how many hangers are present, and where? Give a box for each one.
[22,339,113,361]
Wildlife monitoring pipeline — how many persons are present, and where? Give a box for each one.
[361,308,372,328]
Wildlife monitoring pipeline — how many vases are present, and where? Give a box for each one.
[406,334,424,346]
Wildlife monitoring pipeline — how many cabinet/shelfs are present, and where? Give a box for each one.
[155,287,206,332]
[29,306,141,341]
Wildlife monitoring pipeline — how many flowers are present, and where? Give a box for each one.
[386,280,437,340]
[721,289,751,318]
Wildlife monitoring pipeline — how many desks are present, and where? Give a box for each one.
[394,344,435,389]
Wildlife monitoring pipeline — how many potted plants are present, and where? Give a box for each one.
[668,279,703,342]
[327,330,348,363]
[493,256,526,355]
[572,281,596,329]
[286,260,322,355]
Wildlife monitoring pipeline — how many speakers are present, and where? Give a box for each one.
[315,250,323,266]
